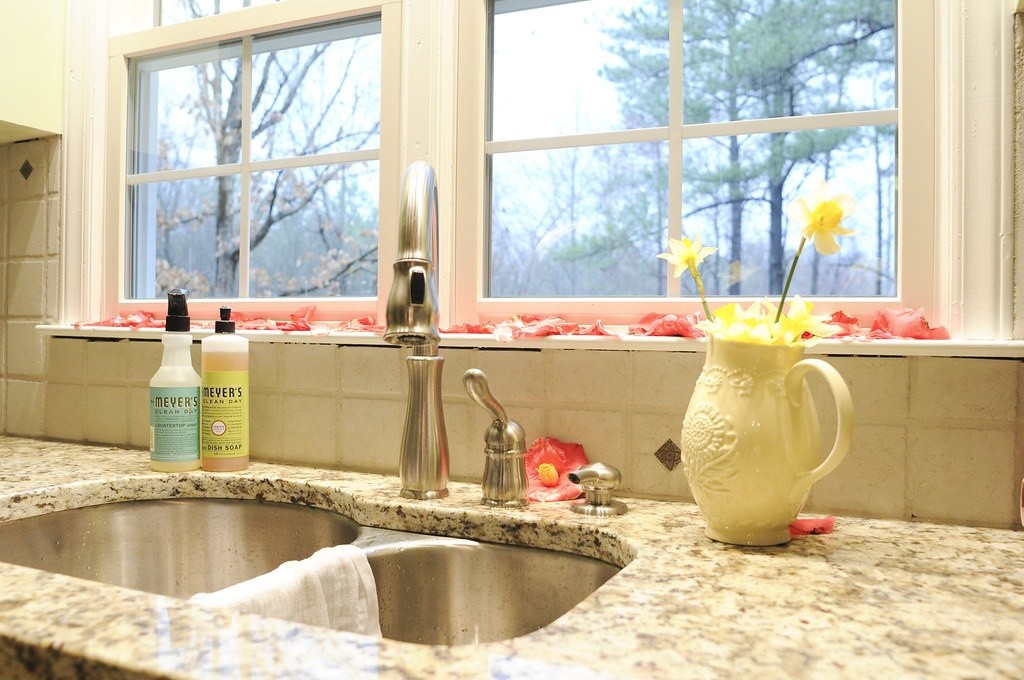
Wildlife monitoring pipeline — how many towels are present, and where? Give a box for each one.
[187,544,384,641]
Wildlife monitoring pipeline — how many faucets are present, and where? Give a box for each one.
[378,157,458,503]
[565,460,630,517]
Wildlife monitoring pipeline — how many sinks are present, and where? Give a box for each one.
[219,539,630,647]
[0,496,366,605]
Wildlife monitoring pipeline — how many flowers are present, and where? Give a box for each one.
[653,162,863,344]
[521,435,591,505]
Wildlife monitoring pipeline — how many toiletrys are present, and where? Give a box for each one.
[145,288,202,473]
[201,306,251,473]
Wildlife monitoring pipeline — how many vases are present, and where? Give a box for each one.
[680,332,857,548]
